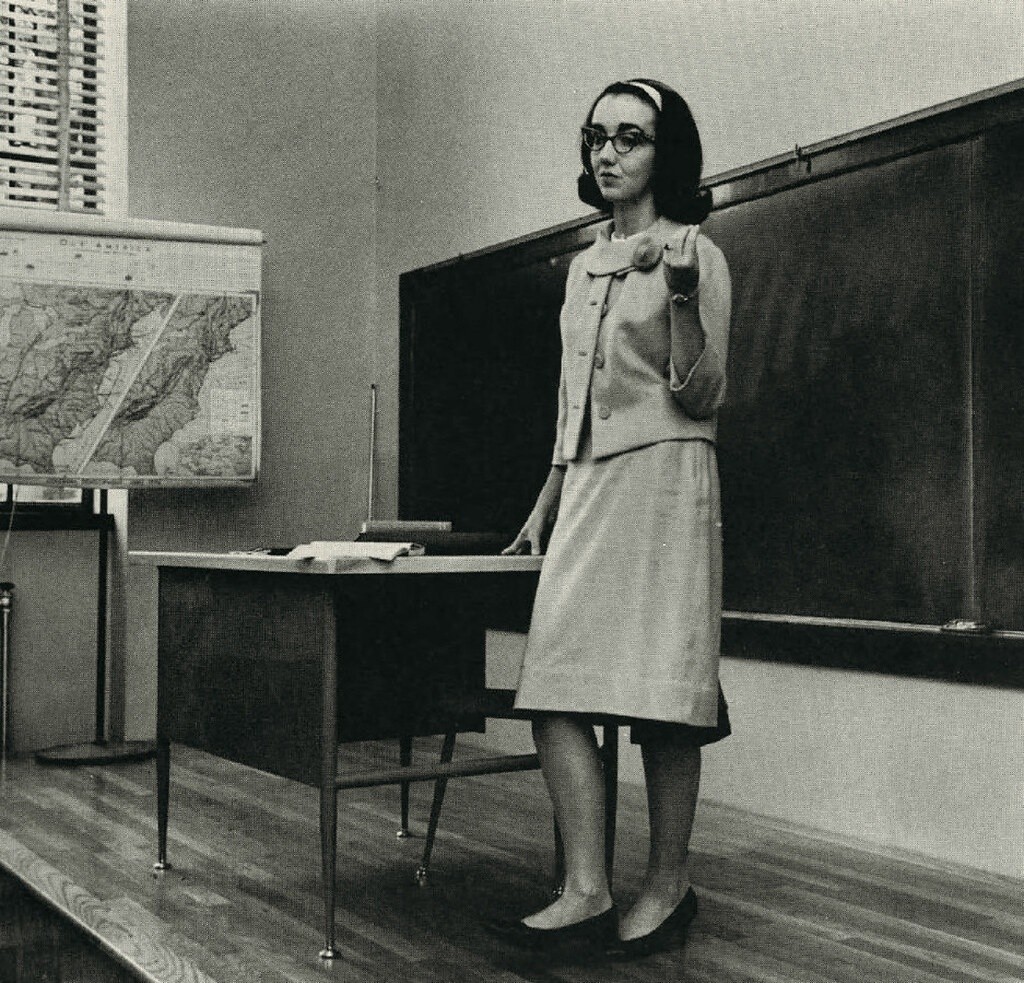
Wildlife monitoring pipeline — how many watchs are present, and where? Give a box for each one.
[668,289,700,306]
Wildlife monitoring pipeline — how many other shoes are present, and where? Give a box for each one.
[484,902,619,946]
[604,886,697,961]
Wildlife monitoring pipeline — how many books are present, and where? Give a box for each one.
[286,540,426,563]
[354,522,514,554]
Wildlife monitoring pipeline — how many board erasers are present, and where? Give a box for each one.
[941,618,991,634]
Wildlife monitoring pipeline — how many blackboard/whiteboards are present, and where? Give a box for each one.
[396,74,1023,691]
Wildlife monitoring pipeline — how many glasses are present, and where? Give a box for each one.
[581,127,656,154]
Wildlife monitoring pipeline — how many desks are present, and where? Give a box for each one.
[127,550,618,959]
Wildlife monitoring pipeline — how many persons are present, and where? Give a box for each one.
[488,78,732,959]
